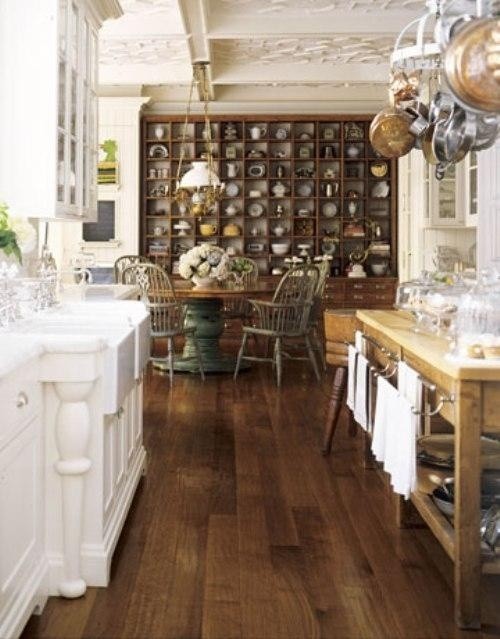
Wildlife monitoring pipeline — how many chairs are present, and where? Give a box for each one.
[115,254,335,388]
[321,310,357,455]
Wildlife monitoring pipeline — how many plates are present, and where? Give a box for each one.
[322,202,337,218]
[415,433,500,463]
[225,184,239,197]
[247,203,264,217]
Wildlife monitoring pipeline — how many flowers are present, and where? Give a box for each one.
[178,242,231,281]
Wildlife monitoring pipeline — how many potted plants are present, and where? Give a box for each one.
[0,200,39,279]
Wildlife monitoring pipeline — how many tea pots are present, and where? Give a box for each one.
[247,125,268,140]
[151,225,166,236]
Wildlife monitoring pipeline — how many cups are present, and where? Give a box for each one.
[200,224,218,236]
[463,335,500,361]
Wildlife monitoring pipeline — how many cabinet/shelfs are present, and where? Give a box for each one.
[402,130,486,231]
[0,0,125,226]
[138,108,401,309]
[0,282,154,639]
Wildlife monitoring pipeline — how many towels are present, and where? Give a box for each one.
[348,330,428,503]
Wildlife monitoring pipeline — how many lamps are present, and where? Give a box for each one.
[175,61,226,215]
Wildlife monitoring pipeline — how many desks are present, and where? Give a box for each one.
[356,308,500,631]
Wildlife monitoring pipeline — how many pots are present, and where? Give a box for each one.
[367,0,500,182]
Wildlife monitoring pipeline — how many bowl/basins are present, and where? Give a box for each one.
[271,244,289,255]
[371,264,387,276]
[429,484,493,519]
